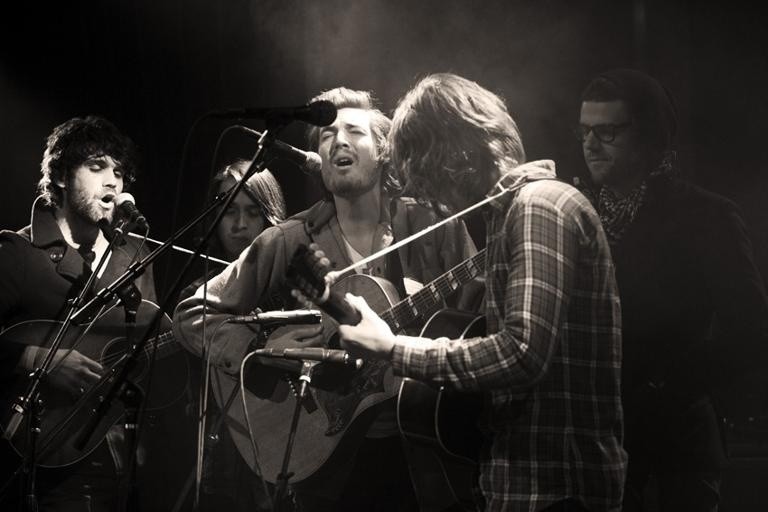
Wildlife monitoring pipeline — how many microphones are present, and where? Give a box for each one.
[217,100,337,126]
[254,348,363,370]
[115,192,150,229]
[240,125,322,171]
[225,309,321,324]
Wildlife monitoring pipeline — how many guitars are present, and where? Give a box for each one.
[0,299,191,471]
[210,246,488,483]
[280,244,486,462]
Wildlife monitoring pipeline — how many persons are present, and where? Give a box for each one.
[178,159,287,511]
[173,87,491,512]
[340,73,628,510]
[2,116,158,511]
[572,71,765,510]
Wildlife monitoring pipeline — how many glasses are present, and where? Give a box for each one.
[581,123,632,143]
